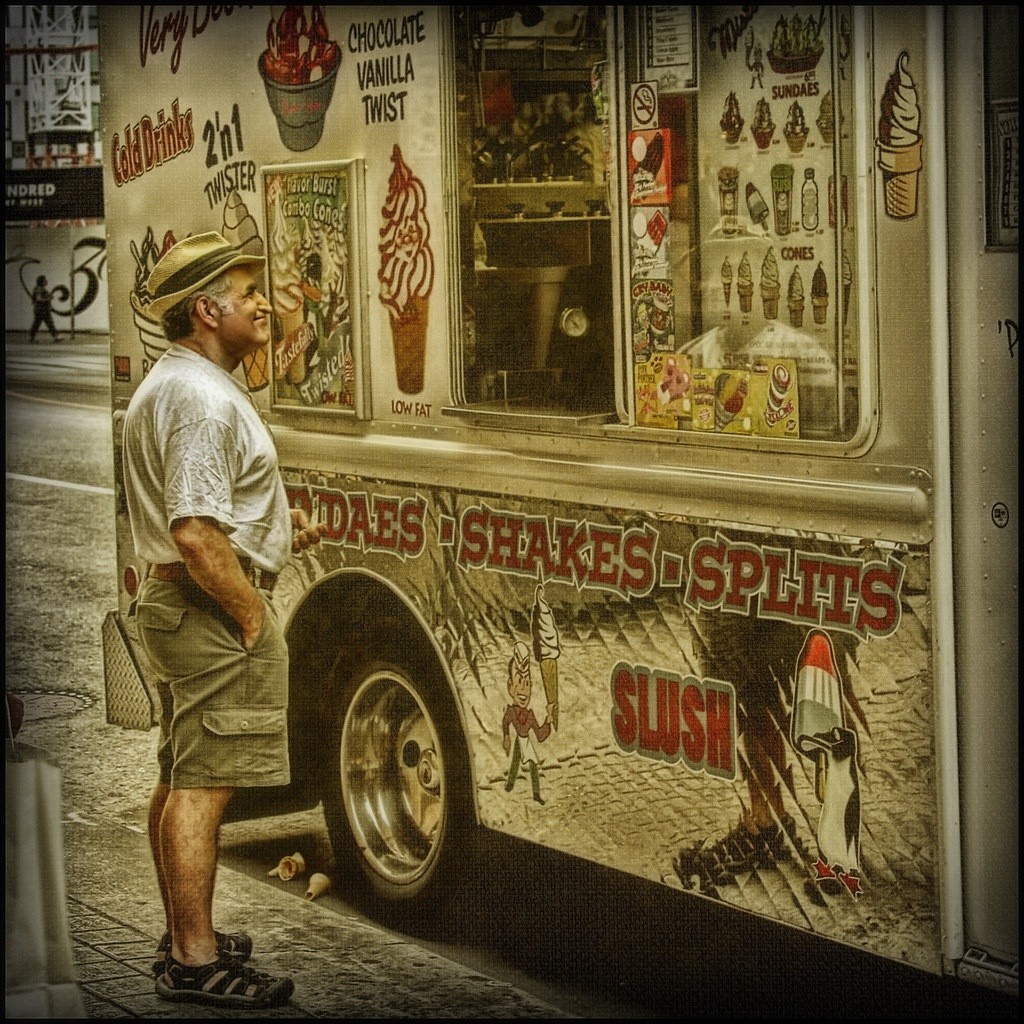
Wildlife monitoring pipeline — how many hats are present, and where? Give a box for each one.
[147,232,267,316]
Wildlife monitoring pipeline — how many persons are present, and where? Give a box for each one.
[116,228,334,1009]
[27,275,64,345]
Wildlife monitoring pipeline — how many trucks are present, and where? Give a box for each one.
[84,0,1024,1009]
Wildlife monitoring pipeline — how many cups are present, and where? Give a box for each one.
[258,39,342,152]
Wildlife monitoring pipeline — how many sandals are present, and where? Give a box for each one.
[151,931,254,972]
[155,951,296,1006]
